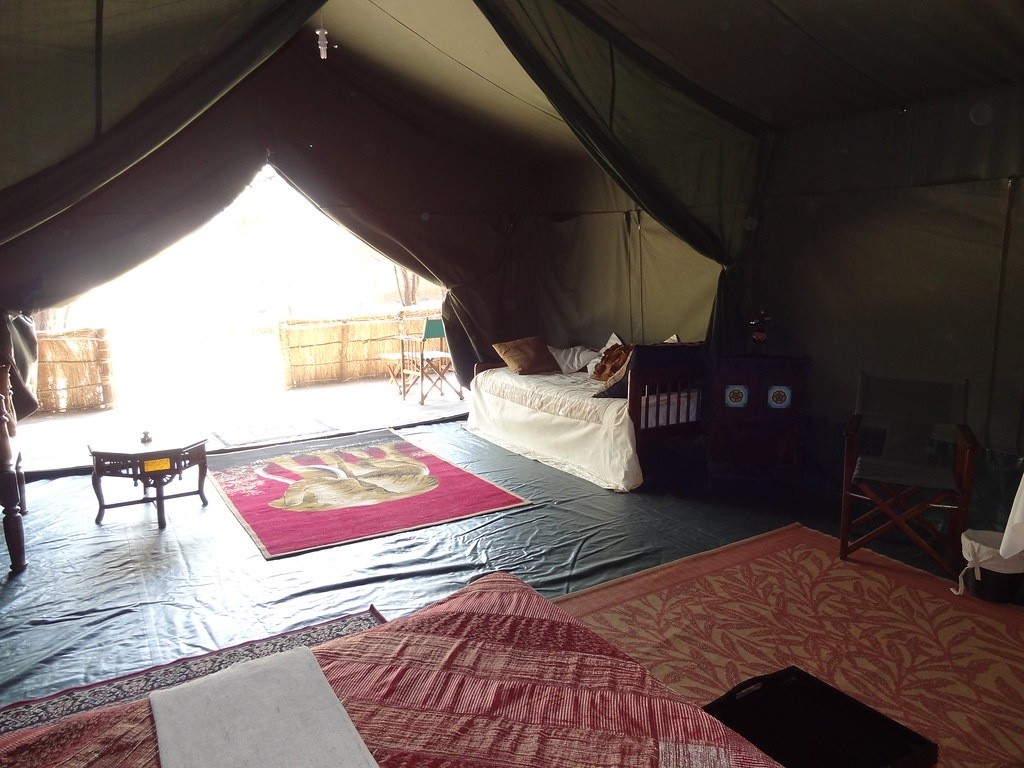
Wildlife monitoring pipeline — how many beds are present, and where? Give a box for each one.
[0,572,786,768]
[462,340,708,493]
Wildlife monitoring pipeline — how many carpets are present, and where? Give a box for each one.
[203,428,532,561]
[0,604,389,733]
[547,522,1024,768]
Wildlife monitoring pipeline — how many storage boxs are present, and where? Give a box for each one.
[700,664,938,768]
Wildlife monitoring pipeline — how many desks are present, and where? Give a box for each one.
[373,351,452,398]
[87,430,207,530]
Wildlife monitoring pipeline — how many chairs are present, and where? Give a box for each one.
[839,372,980,584]
[394,318,464,404]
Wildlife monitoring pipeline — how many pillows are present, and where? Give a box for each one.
[492,336,563,375]
[547,344,600,374]
[586,332,686,398]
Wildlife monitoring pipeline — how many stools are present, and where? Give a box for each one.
[961,528,1024,604]
